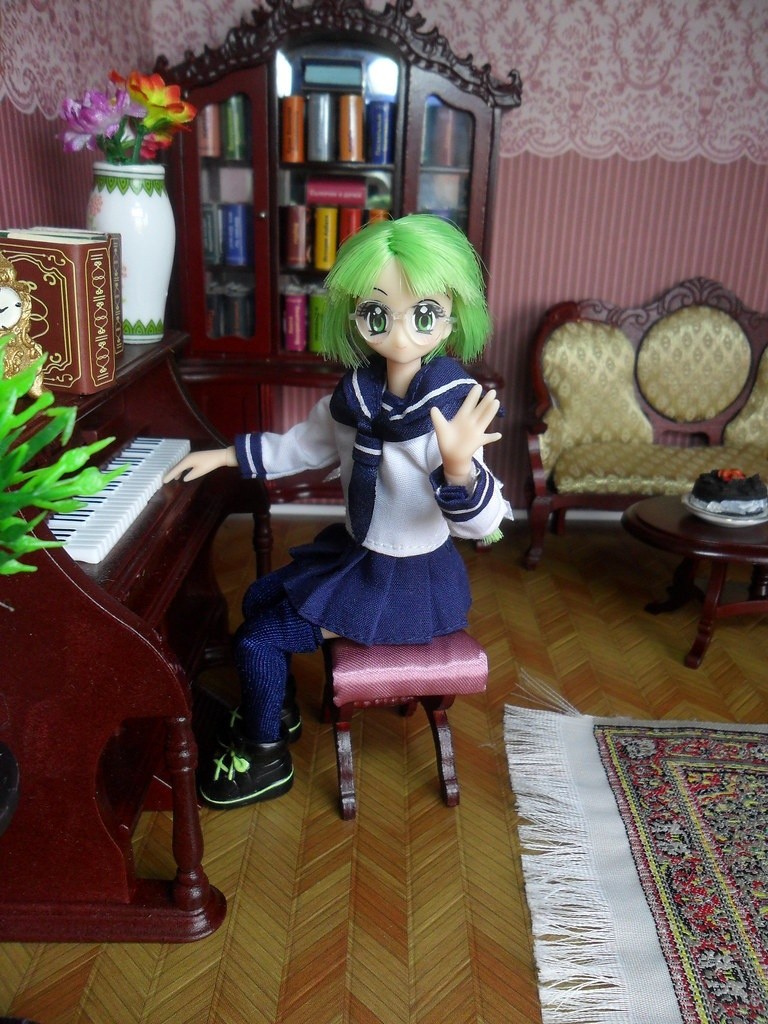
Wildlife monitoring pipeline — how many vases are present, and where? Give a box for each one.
[85,161,177,346]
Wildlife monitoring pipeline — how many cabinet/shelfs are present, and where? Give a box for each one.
[151,0,524,506]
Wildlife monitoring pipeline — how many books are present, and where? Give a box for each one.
[0,225,126,397]
[192,90,465,353]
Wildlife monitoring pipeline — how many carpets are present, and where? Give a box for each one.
[499,666,765,1022]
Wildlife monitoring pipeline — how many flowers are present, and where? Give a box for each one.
[55,71,199,166]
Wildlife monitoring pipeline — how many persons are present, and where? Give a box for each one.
[160,212,515,811]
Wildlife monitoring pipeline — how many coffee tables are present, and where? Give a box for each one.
[619,494,768,668]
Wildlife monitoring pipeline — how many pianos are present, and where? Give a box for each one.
[0,327,274,944]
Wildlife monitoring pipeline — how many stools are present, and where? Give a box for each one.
[318,629,489,822]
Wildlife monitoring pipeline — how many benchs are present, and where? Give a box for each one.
[521,276,768,572]
